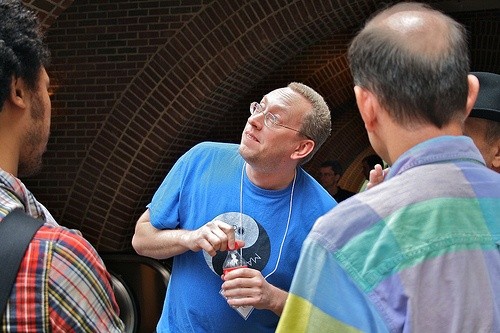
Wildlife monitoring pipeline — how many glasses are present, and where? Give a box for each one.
[316,172,335,179]
[250,102,316,145]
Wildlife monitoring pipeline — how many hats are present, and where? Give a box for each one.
[467,71,500,111]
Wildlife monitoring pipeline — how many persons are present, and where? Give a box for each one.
[361,154,385,190]
[367,71,500,187]
[319,159,357,204]
[0,0,133,333]
[131,83,338,333]
[276,2,500,333]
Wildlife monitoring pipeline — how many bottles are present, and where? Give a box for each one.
[222,241,248,310]
